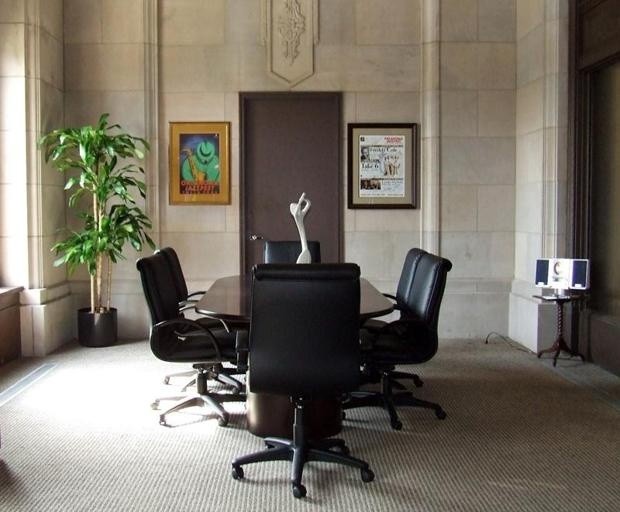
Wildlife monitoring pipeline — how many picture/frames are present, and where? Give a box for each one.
[346,122,418,209]
[169,121,230,206]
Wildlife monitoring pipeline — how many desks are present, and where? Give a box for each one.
[534,294,588,367]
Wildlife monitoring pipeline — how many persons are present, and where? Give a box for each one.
[361,146,399,190]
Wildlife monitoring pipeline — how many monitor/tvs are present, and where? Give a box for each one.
[532,257,590,291]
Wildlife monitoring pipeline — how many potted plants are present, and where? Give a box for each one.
[36,110,157,347]
